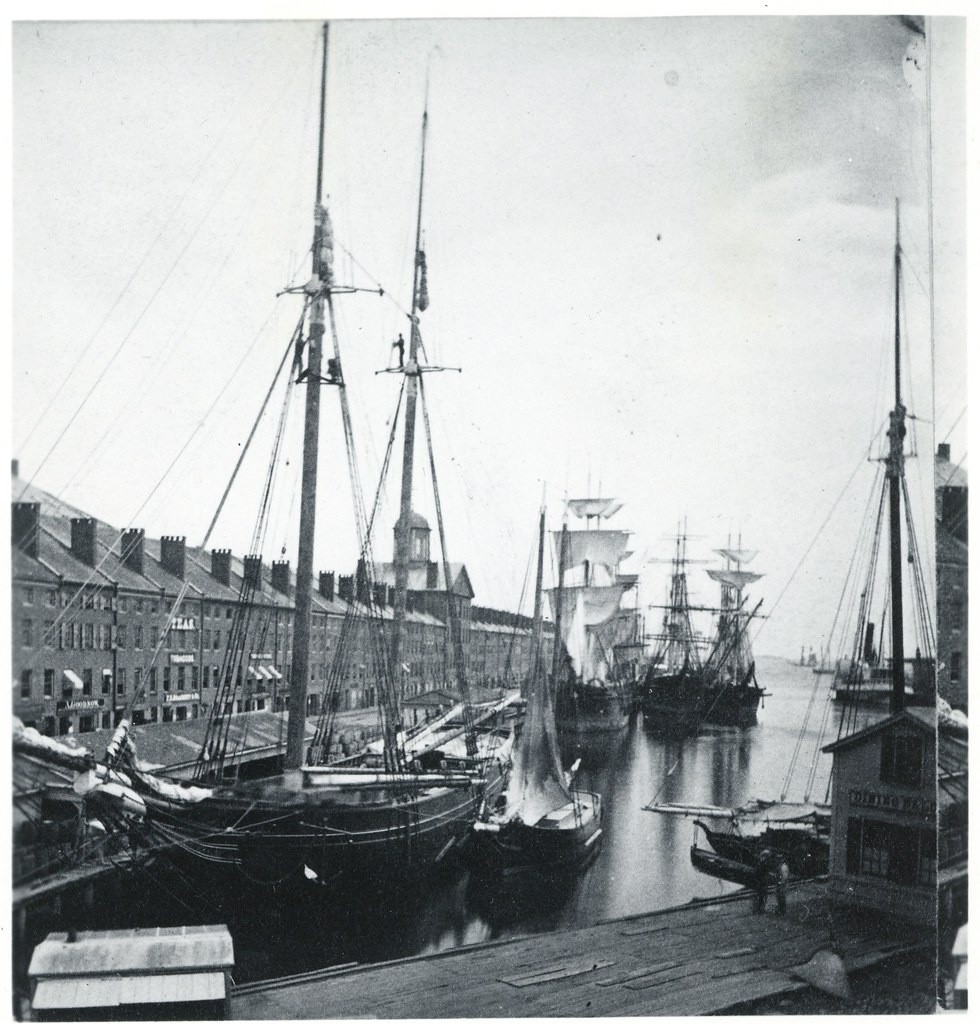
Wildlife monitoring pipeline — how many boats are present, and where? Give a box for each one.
[830,664,915,706]
[690,798,827,888]
[649,667,710,730]
[70,721,514,887]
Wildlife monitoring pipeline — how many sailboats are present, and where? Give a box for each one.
[552,493,648,732]
[710,529,766,730]
[508,620,619,878]
